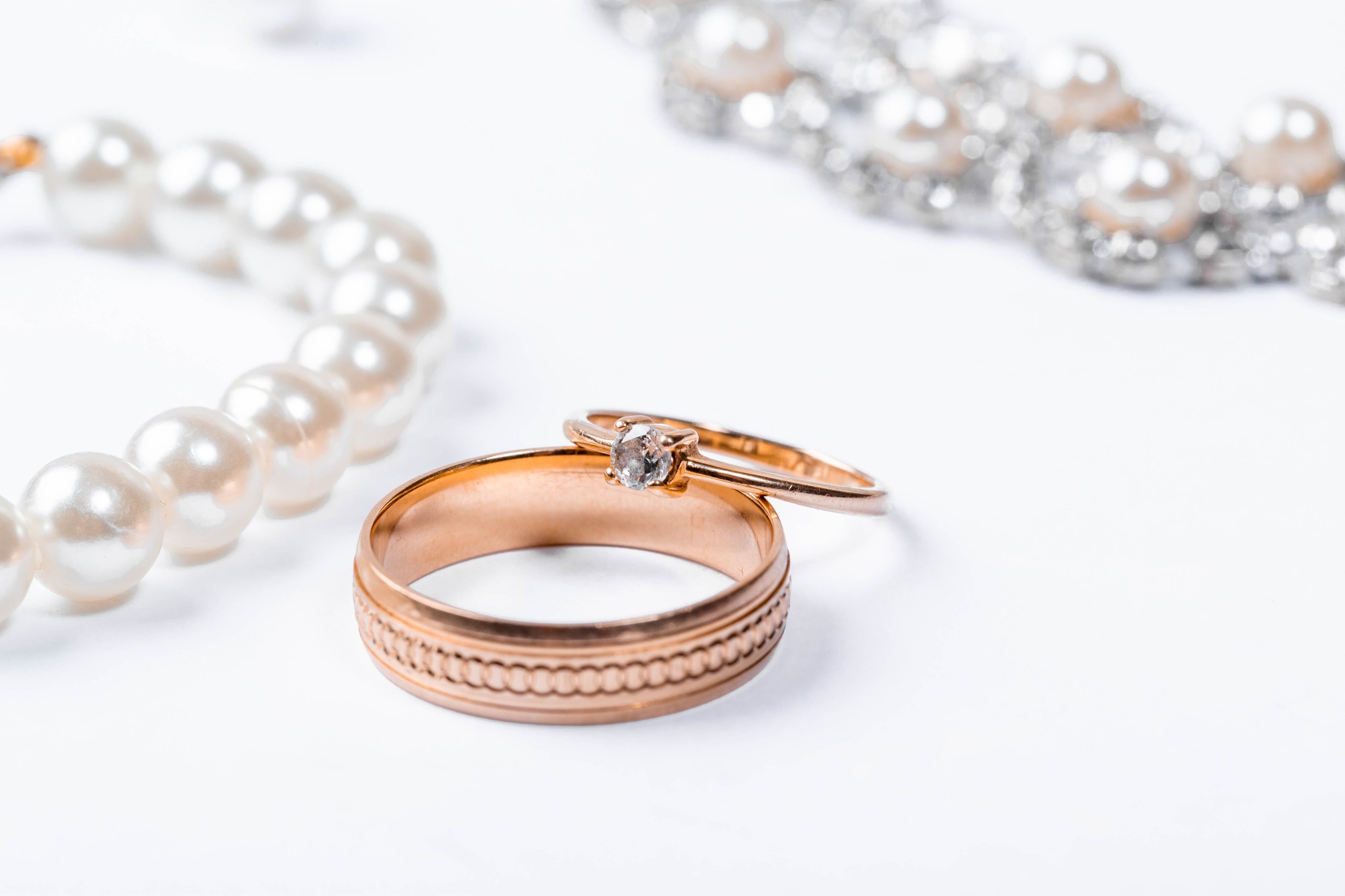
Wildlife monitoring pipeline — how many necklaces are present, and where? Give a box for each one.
[0,115,451,630]
[593,0,1345,308]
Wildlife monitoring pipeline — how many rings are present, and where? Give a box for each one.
[352,446,792,726]
[564,409,888,517]
